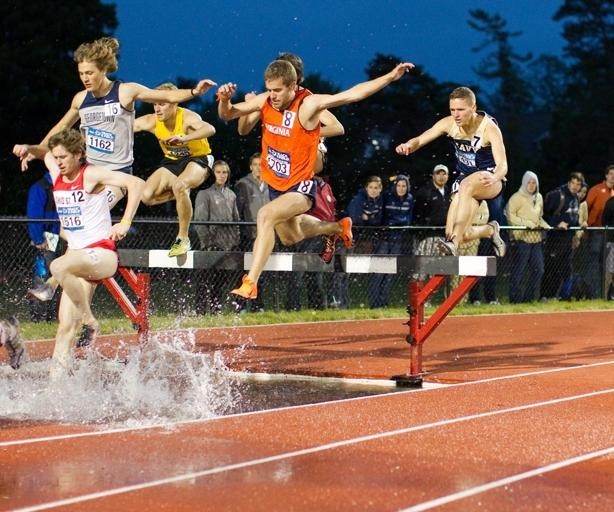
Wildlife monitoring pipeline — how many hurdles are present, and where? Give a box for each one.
[85,247,498,388]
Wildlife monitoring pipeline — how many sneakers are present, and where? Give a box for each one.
[436,238,457,256]
[168,235,192,258]
[72,320,100,359]
[229,279,257,299]
[26,283,55,302]
[472,300,480,305]
[487,220,507,258]
[489,301,501,306]
[338,217,353,251]
[321,234,338,263]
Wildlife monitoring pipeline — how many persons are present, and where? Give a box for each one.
[26,170,68,323]
[216,60,413,301]
[284,164,453,310]
[470,167,613,305]
[133,83,217,257]
[233,151,271,313]
[396,87,508,258]
[193,161,241,316]
[21,35,218,303]
[238,52,346,263]
[12,129,146,383]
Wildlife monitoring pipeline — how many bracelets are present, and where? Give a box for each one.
[119,219,132,226]
[215,93,232,103]
[190,88,196,96]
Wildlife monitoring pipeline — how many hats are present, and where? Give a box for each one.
[433,164,449,174]
